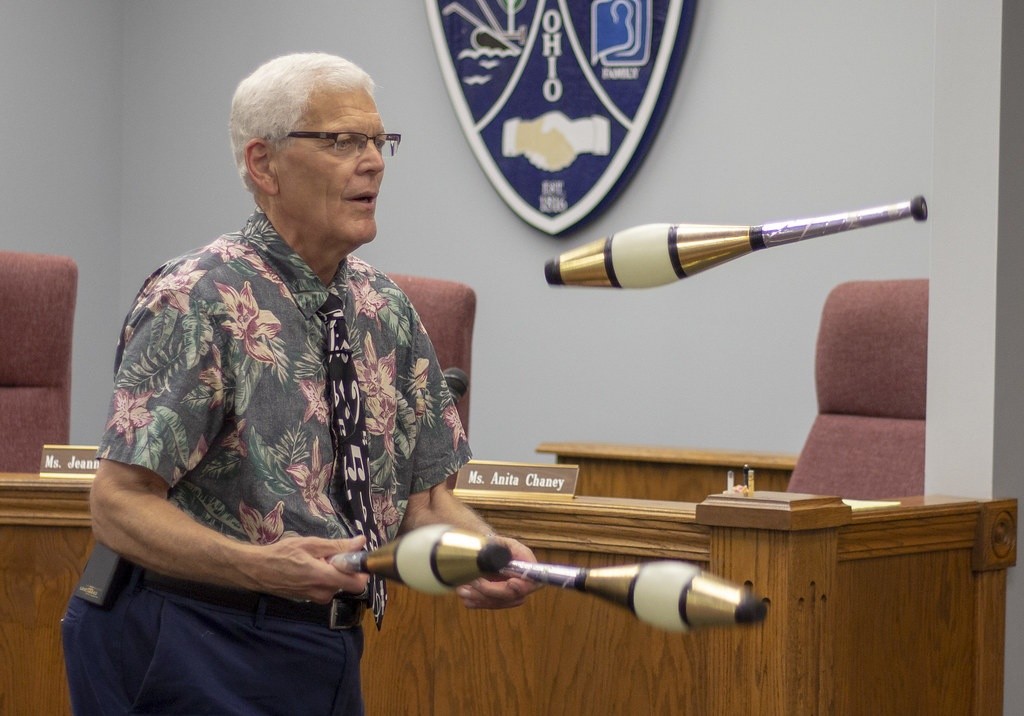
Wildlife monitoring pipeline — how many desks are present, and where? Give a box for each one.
[0,470,1018,716]
[533,441,797,504]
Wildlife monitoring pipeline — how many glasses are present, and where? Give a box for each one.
[264,132,401,157]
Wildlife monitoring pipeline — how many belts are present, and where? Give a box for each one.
[119,558,367,630]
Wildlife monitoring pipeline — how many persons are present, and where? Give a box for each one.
[55,49,544,715]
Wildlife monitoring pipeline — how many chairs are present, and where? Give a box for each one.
[378,269,477,452]
[0,251,81,470]
[781,274,926,493]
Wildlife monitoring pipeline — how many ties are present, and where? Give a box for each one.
[316,293,389,631]
